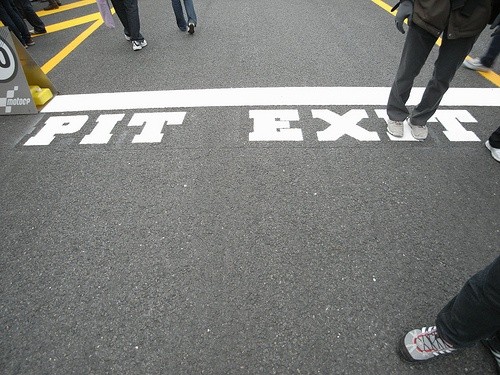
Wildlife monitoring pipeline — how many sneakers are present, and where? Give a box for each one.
[384,113,405,137]
[408,117,428,140]
[484,140,500,161]
[131,39,148,51]
[482,336,500,368]
[124,34,132,41]
[396,325,480,364]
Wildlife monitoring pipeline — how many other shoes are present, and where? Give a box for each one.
[57,1,61,6]
[22,37,35,46]
[29,30,46,34]
[463,58,489,72]
[187,23,195,34]
[21,40,28,48]
[43,1,59,10]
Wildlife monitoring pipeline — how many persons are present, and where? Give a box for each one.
[386,0,500,139]
[111,0,148,51]
[0,0,63,49]
[464,28,500,72]
[397,254,500,372]
[171,0,196,34]
[485,126,500,163]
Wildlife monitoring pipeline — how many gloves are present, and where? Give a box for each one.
[489,15,500,37]
[391,1,414,34]
